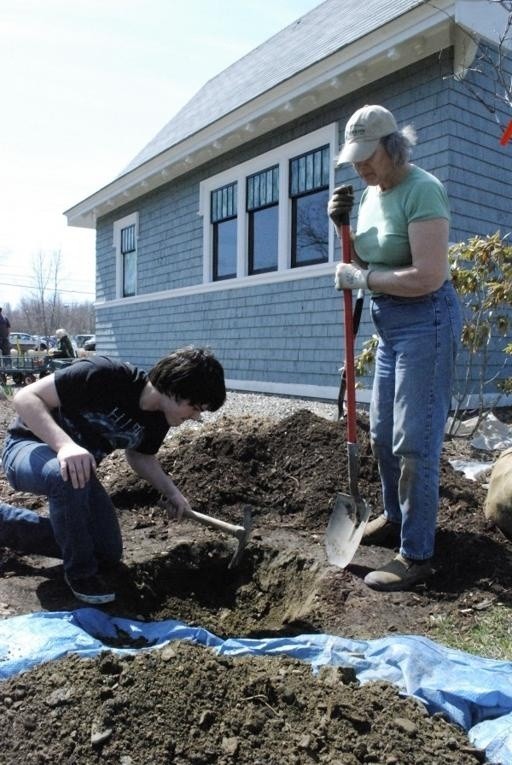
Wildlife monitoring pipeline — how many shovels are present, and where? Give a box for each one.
[325,195,370,569]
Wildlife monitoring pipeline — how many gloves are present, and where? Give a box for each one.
[327,185,354,228]
[334,260,373,291]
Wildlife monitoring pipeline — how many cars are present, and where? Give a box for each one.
[32,335,94,349]
[84,336,97,352]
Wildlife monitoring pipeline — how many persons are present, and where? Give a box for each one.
[48,328,75,358]
[0,306,11,354]
[322,101,465,590]
[1,339,233,612]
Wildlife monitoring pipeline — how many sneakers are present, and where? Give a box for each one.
[361,514,401,544]
[64,572,115,603]
[364,554,432,591]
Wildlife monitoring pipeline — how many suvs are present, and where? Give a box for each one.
[8,332,52,352]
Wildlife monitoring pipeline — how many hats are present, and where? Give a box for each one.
[336,104,398,168]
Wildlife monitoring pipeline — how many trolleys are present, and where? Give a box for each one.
[0,354,52,387]
